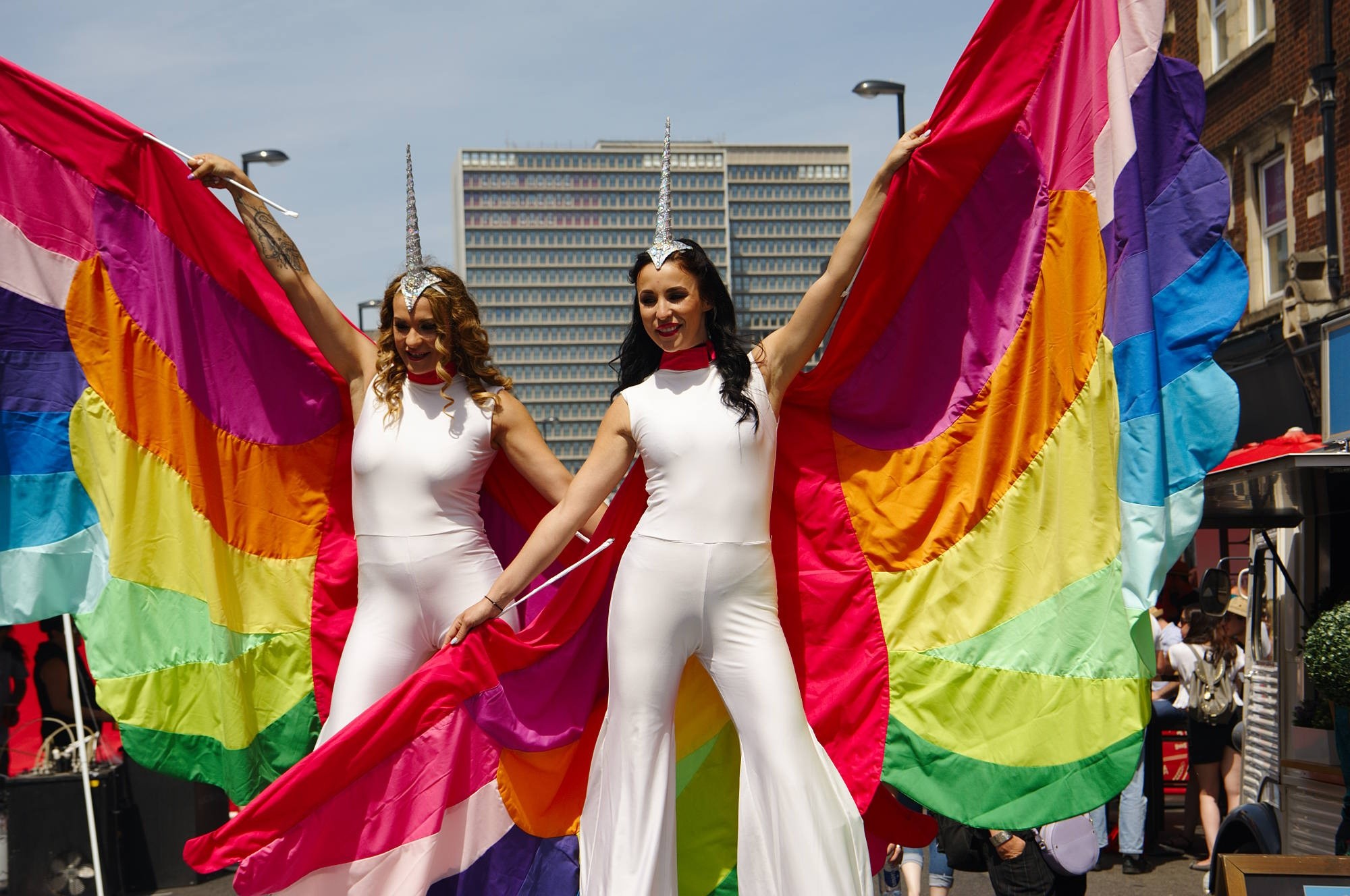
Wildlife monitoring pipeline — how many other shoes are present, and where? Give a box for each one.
[1167,831,1194,842]
[1189,860,1211,870]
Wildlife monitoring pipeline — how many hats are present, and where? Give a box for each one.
[1227,597,1249,618]
[1150,607,1163,618]
[1168,559,1192,577]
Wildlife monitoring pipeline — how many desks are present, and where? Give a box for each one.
[4,765,156,896]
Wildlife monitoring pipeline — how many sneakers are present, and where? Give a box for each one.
[1122,853,1152,873]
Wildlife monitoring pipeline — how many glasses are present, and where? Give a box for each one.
[1180,617,1190,626]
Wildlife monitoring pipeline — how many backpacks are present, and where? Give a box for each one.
[1178,642,1243,727]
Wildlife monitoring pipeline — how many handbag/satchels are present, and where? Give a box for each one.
[1034,815,1100,877]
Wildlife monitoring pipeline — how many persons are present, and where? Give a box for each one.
[0,614,117,776]
[885,588,1248,896]
[181,152,608,752]
[443,122,936,896]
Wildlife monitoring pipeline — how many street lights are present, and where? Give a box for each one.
[852,79,906,141]
[242,148,290,178]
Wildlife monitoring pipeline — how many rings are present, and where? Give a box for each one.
[895,844,903,850]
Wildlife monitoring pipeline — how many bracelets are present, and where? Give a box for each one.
[484,596,503,612]
[989,830,1013,847]
